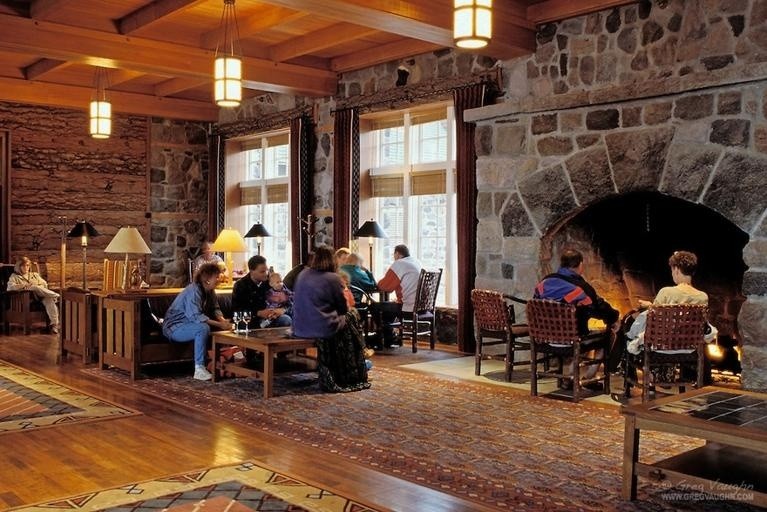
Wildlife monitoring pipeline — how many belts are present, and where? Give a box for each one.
[242,311,252,333]
[233,311,242,333]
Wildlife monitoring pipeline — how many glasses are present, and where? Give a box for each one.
[214,0,242,107]
[244,221,273,256]
[353,218,389,274]
[89,66,112,139]
[209,229,249,289]
[66,221,101,292]
[103,227,152,291]
[453,0,493,49]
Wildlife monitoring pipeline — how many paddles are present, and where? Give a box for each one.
[81,365,767,512]
[0,358,134,432]
[5,462,382,512]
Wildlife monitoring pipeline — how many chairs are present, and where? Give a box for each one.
[562,376,603,392]
[193,368,212,380]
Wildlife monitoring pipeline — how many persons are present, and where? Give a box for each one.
[162,263,233,381]
[192,240,228,281]
[609,250,709,390]
[291,245,375,392]
[534,248,619,391]
[231,246,377,359]
[7,256,60,334]
[369,245,430,348]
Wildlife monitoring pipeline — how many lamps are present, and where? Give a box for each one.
[625,305,707,402]
[349,284,380,348]
[382,269,443,353]
[471,289,549,382]
[526,297,611,403]
